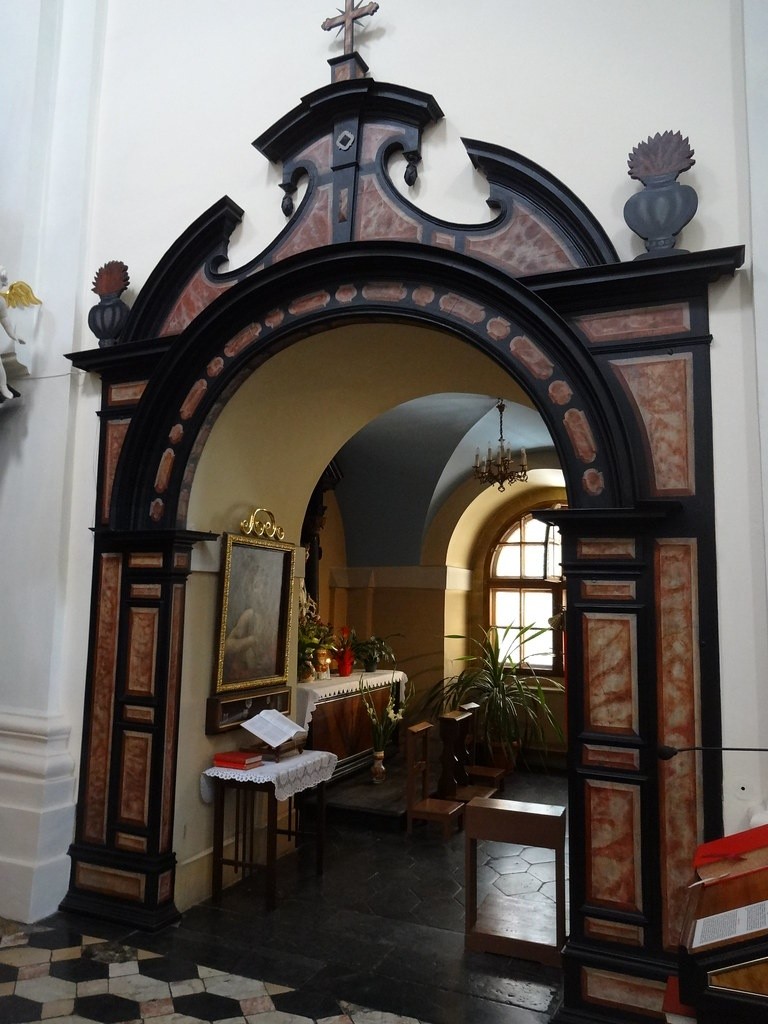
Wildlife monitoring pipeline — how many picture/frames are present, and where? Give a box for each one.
[214,507,296,694]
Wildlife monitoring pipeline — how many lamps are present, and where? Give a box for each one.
[473,397,529,493]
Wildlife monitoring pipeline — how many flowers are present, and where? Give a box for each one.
[359,661,414,750]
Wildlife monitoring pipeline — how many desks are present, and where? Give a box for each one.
[200,750,338,913]
[297,668,409,766]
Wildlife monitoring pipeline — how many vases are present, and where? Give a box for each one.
[369,748,386,783]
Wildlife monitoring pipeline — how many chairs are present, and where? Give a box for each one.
[459,702,505,800]
[406,721,465,844]
[437,711,498,831]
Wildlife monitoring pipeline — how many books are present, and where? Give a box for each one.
[213,751,263,771]
[239,708,307,748]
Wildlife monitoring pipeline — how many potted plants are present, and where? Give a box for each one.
[416,617,565,780]
[332,625,359,676]
[355,634,397,672]
[314,622,339,680]
[298,625,327,683]
